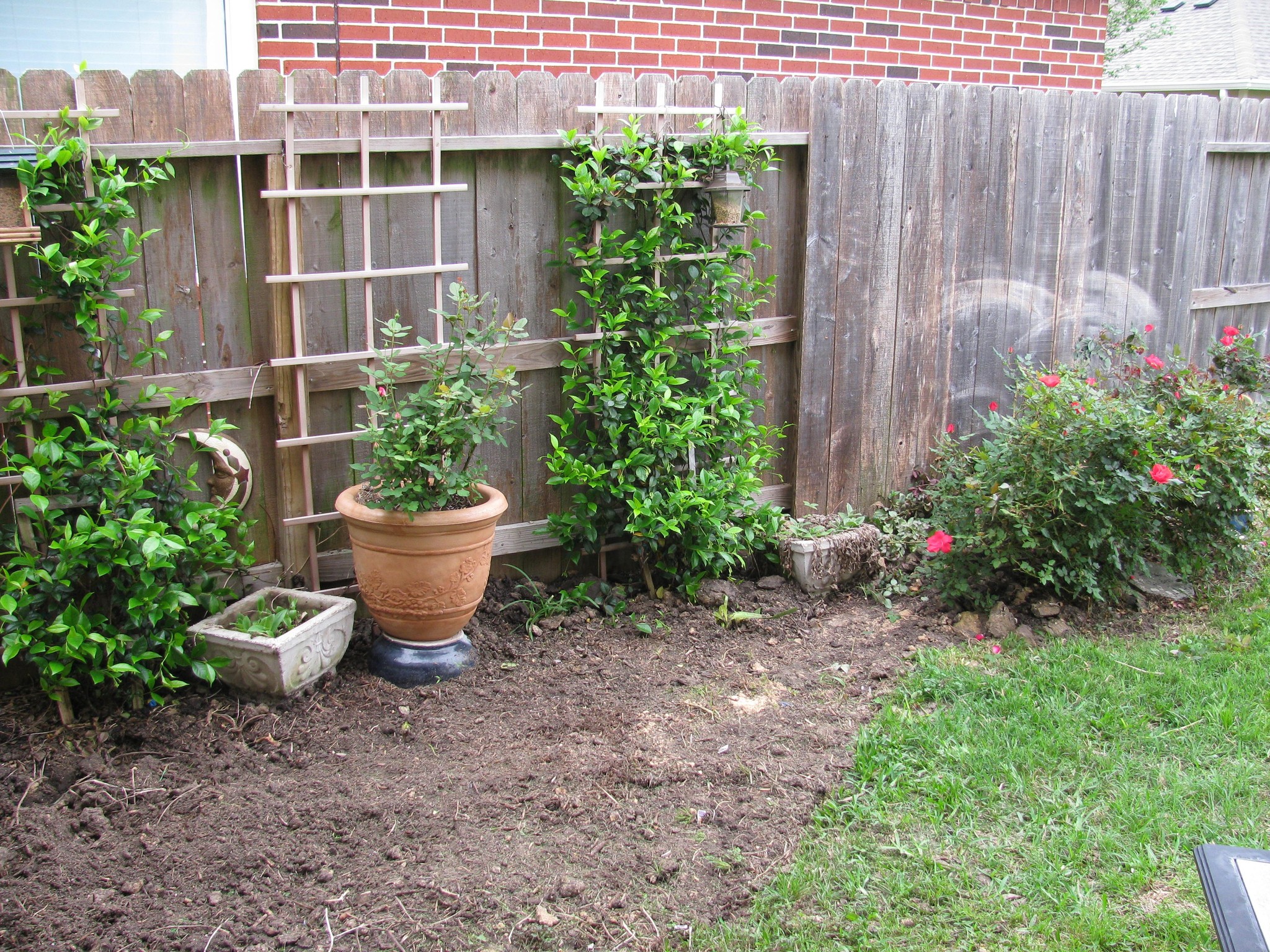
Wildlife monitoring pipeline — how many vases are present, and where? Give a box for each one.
[333,483,510,692]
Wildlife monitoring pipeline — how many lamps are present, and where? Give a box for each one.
[703,163,749,231]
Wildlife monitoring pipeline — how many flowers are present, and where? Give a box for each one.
[355,283,529,512]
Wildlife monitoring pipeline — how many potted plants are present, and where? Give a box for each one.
[782,512,877,595]
[188,586,356,702]
